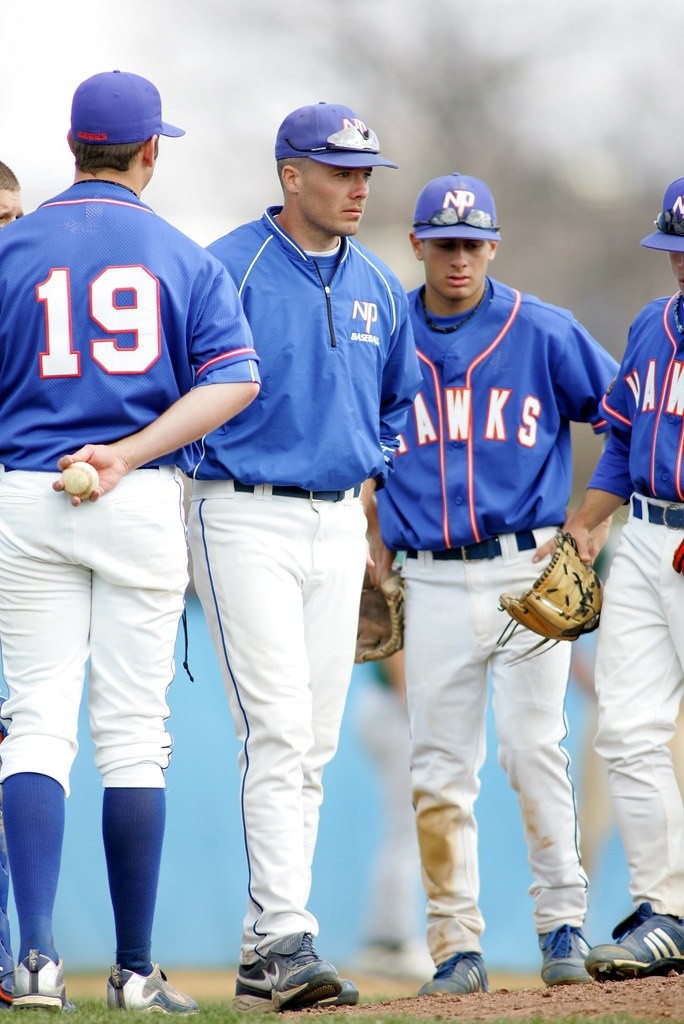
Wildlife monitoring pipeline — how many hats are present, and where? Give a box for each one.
[640,177,684,252]
[276,102,400,170]
[412,173,503,241]
[71,71,185,144]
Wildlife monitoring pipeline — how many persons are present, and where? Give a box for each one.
[185,102,425,1010]
[532,173,684,985]
[0,163,27,1009]
[0,68,264,1015]
[367,172,635,1000]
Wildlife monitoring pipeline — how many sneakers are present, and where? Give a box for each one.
[584,902,684,982]
[263,930,343,1012]
[233,958,358,1014]
[107,964,200,1018]
[537,925,596,987]
[11,949,77,1012]
[417,951,489,999]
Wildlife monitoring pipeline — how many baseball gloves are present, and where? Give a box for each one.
[352,565,405,664]
[492,528,605,669]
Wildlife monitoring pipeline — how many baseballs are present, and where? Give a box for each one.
[62,461,100,500]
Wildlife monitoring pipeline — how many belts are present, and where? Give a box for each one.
[632,496,684,529]
[406,531,536,563]
[233,478,361,502]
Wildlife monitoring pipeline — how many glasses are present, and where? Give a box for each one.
[285,127,381,154]
[414,207,501,231]
[655,209,684,236]
